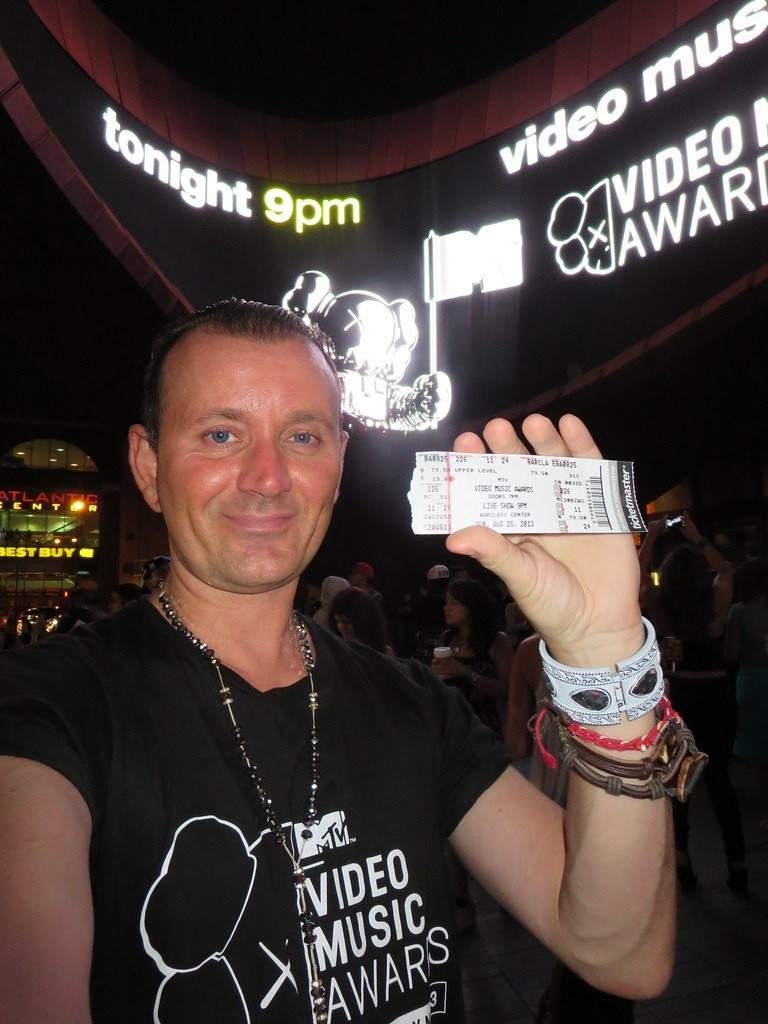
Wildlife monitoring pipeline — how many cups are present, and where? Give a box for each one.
[433,647,451,679]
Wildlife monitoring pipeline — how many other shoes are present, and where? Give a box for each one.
[677,873,698,897]
[725,871,751,898]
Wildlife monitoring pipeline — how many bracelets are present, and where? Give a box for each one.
[539,616,665,726]
[467,671,480,681]
[698,536,707,550]
[569,722,676,782]
[562,718,711,803]
[535,697,673,769]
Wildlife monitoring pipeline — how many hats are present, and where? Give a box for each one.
[347,563,374,580]
[141,556,170,579]
[450,565,470,579]
[427,564,449,580]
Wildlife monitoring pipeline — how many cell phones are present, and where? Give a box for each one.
[664,515,685,529]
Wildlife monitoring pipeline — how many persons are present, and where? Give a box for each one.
[0,298,768,1024]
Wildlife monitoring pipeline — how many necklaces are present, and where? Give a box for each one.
[157,577,327,1024]
[452,643,464,654]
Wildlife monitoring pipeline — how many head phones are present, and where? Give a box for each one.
[142,556,169,581]
